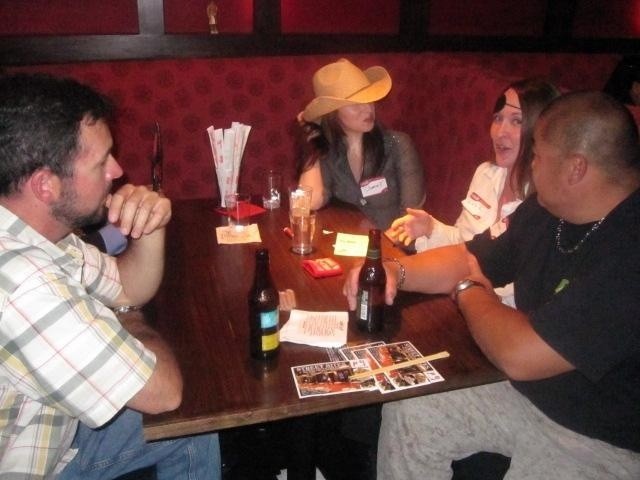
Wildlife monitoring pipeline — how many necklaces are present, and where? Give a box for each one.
[555,215,605,256]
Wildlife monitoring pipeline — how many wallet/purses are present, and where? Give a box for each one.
[301,257,342,278]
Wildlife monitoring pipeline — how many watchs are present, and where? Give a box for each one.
[454,278,485,298]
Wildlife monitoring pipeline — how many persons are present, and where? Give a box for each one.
[390,74,563,254]
[0,73,223,480]
[605,55,640,132]
[294,57,428,255]
[342,88,639,480]
[75,192,130,258]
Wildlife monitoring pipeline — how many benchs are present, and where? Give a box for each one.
[11,56,636,226]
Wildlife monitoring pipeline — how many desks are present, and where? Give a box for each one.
[141,199,514,480]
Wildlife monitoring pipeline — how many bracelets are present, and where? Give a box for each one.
[113,305,141,317]
[393,257,406,289]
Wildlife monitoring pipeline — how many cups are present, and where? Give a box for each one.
[287,186,313,226]
[260,170,283,210]
[224,191,254,236]
[290,210,315,254]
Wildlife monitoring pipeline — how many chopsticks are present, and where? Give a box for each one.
[205,123,253,207]
[350,349,448,380]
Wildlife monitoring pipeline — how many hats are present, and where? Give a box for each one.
[296,58,392,126]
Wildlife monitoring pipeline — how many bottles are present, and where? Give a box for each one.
[354,229,387,331]
[248,249,282,360]
